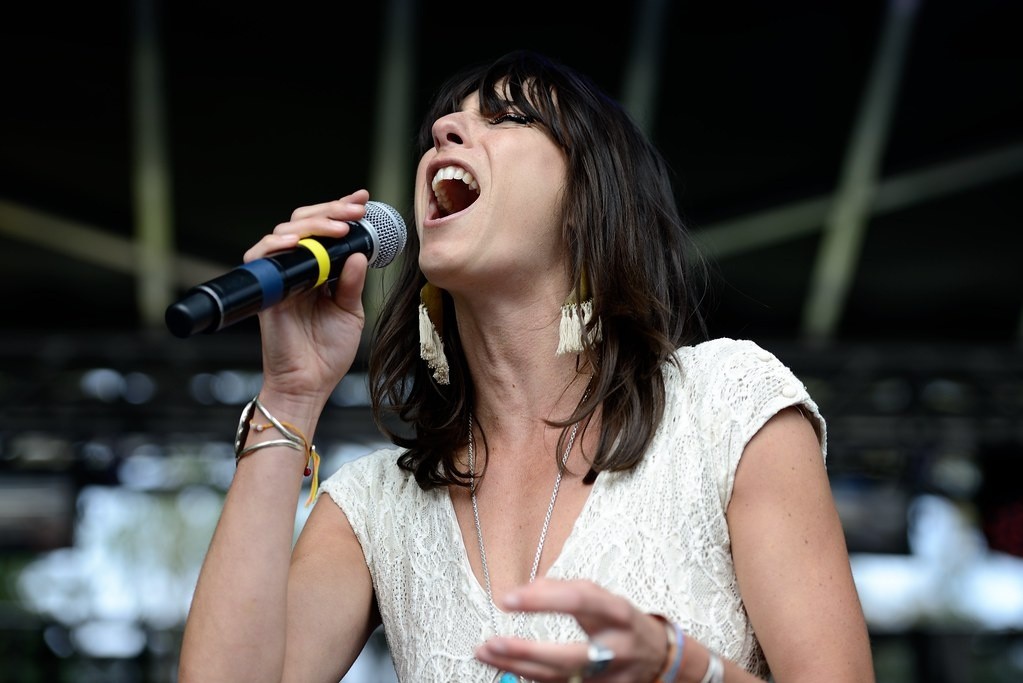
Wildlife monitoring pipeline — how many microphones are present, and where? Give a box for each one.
[166,199,406,339]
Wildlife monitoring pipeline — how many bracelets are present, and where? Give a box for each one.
[701,649,725,683]
[645,611,683,682]
[231,395,322,508]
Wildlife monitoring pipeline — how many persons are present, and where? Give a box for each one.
[176,50,878,683]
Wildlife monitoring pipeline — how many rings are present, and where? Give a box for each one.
[567,671,584,683]
[578,638,616,678]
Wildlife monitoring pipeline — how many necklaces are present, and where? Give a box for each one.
[466,353,601,683]
